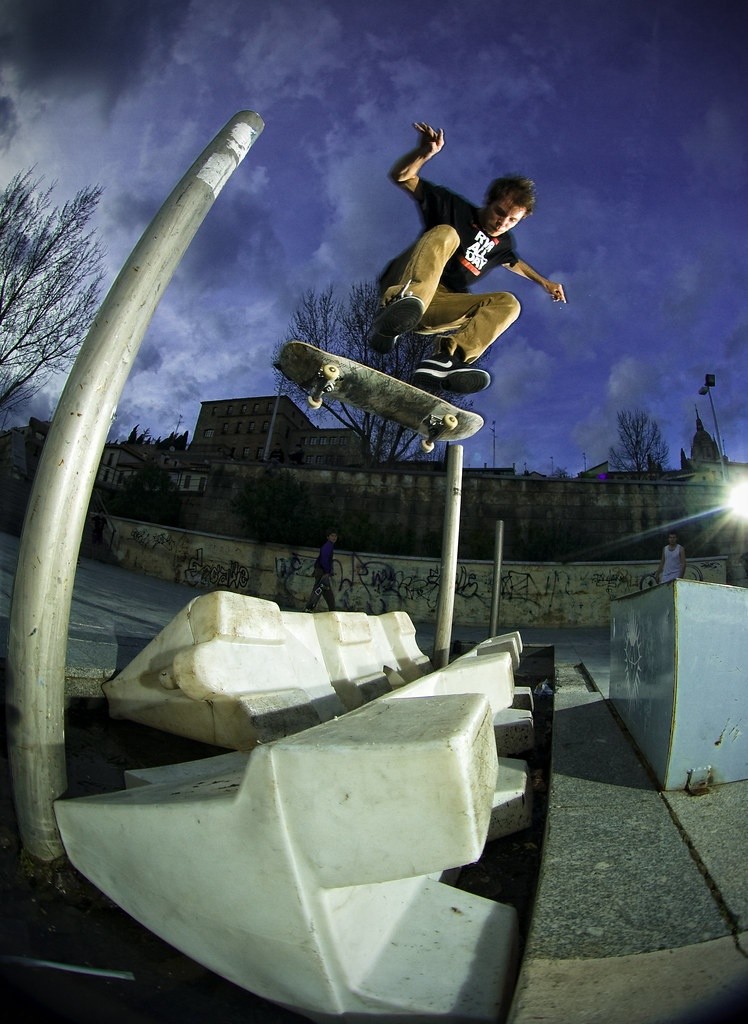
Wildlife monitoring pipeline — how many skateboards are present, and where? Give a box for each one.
[271,335,488,456]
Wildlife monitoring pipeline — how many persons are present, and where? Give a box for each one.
[364,123,569,397]
[656,531,686,584]
[87,509,108,553]
[304,526,337,613]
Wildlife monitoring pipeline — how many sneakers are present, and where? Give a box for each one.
[370,278,425,354]
[414,348,491,393]
